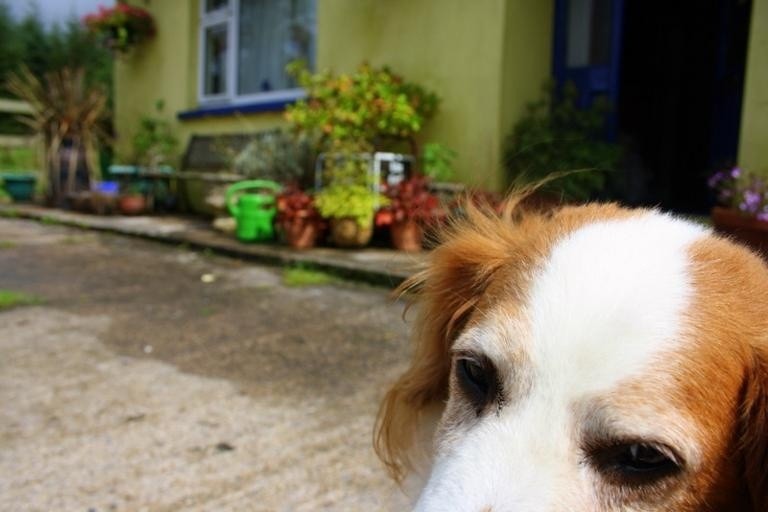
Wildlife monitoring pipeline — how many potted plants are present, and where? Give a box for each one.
[312,174,391,246]
[287,59,439,183]
[373,179,447,254]
[270,186,326,253]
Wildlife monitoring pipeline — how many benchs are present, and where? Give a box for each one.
[142,132,283,217]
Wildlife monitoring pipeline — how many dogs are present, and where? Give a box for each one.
[370,162,768,512]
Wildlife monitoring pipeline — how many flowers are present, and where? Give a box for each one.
[709,167,768,224]
